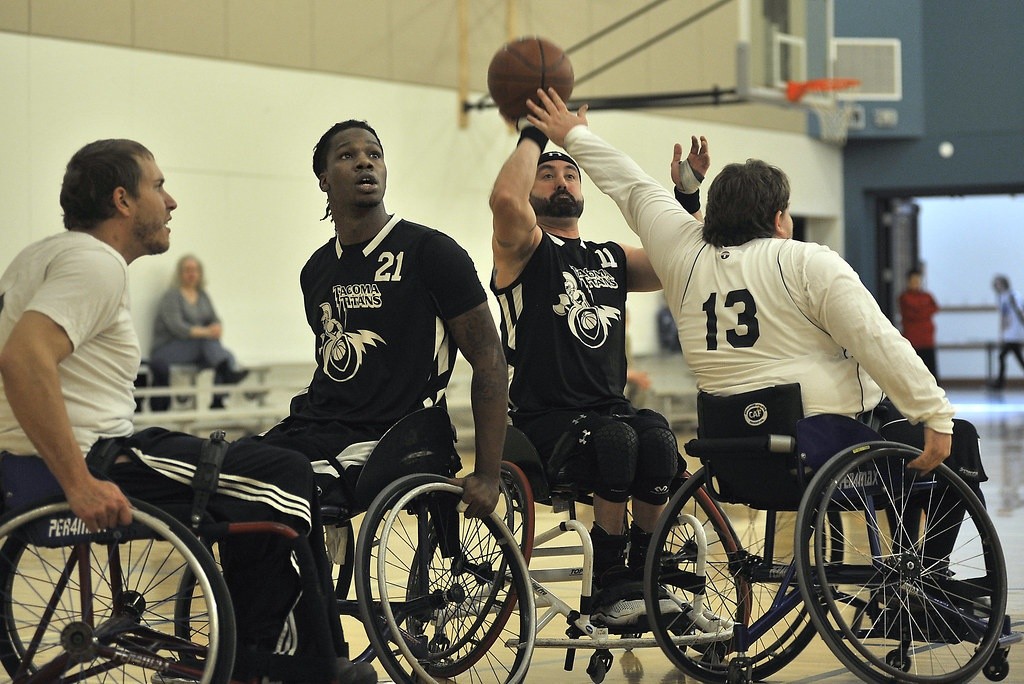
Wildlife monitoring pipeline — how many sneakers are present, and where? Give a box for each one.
[589,565,688,634]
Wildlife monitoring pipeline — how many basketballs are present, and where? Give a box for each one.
[486,35,575,118]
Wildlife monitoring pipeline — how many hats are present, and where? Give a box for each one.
[537,151,580,178]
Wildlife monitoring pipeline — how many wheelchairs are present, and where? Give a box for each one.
[0,417,1024,684]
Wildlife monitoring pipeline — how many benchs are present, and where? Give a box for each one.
[132,386,271,406]
[137,360,274,385]
[131,407,279,429]
[649,385,699,431]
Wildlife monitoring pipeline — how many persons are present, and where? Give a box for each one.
[0,139,377,684]
[488,113,710,628]
[624,310,648,409]
[658,291,683,353]
[149,255,248,408]
[992,275,1024,388]
[215,119,510,684]
[899,268,938,383]
[527,87,997,617]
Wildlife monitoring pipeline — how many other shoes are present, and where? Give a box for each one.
[334,657,378,684]
